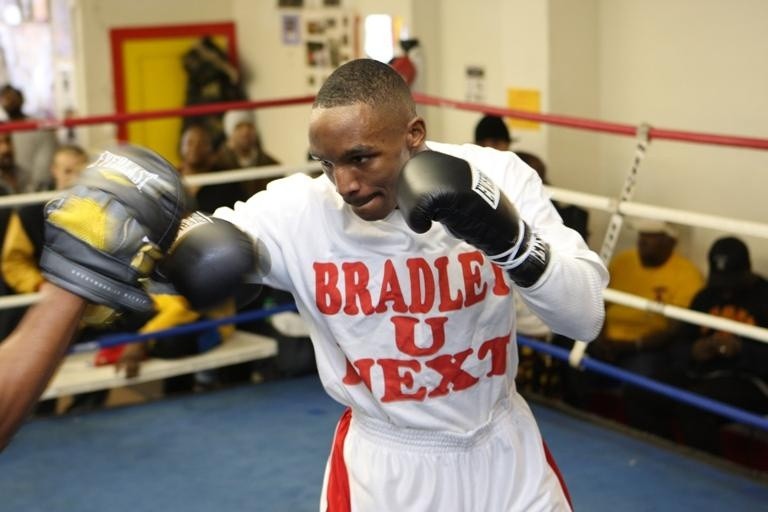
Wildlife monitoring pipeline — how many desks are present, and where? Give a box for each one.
[36,330,279,402]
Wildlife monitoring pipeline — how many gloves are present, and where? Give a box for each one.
[396,151,553,290]
[39,144,185,315]
[157,211,263,313]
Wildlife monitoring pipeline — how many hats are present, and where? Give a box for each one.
[222,110,252,136]
[634,218,678,239]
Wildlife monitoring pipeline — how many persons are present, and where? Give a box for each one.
[157,59,609,512]
[0,143,187,454]
